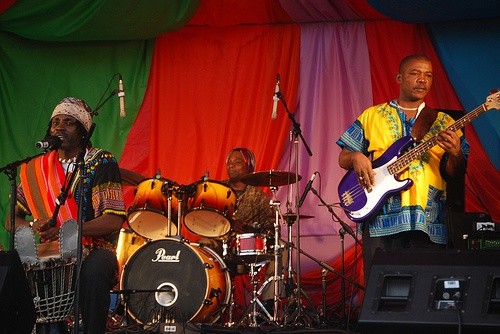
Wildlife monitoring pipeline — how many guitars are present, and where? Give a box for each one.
[338,89,500,224]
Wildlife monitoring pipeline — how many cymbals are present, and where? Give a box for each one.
[239,171,303,187]
[267,213,316,221]
[119,166,145,186]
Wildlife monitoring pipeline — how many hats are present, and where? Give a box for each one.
[50,97,92,133]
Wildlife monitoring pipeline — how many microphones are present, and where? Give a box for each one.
[118,75,126,118]
[271,79,280,119]
[298,172,316,207]
[36,135,61,151]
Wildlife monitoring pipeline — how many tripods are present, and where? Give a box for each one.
[213,99,364,328]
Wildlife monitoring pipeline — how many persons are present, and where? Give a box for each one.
[5,96,126,334]
[335,54,470,252]
[200,147,281,324]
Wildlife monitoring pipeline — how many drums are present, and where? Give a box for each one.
[119,238,232,327]
[23,241,78,324]
[229,233,274,262]
[110,228,149,290]
[183,179,238,237]
[126,177,187,240]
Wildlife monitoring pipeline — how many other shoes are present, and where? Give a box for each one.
[256,306,274,324]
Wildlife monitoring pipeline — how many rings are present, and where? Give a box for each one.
[358,177,363,180]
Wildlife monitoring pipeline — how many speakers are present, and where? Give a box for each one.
[357,248,500,334]
